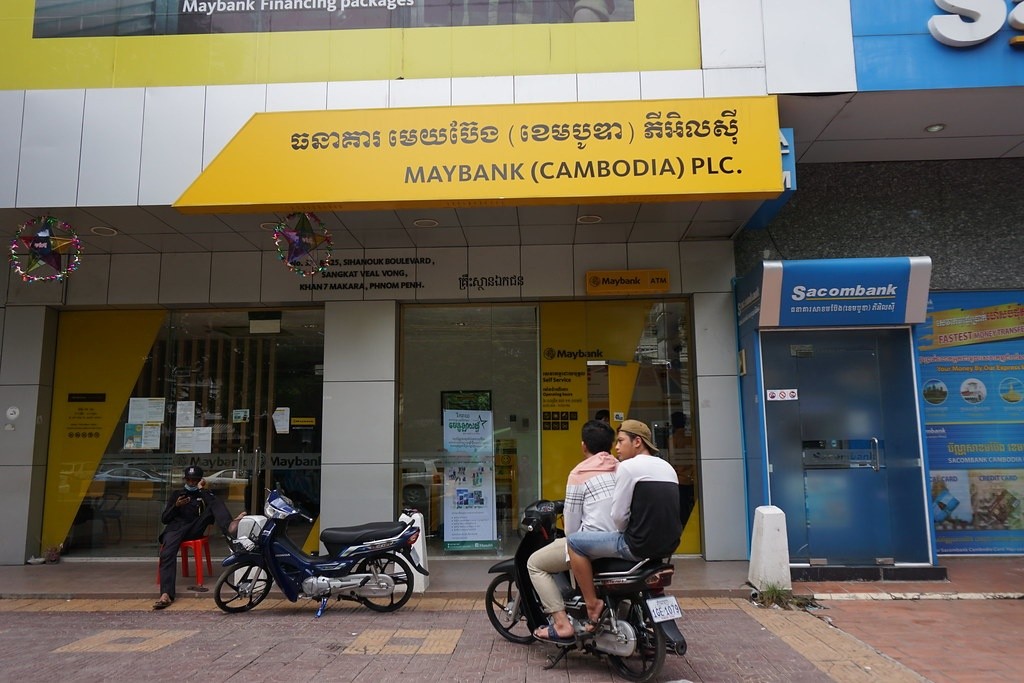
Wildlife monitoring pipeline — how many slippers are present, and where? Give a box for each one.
[186,585,207,592]
[153,599,170,607]
[586,608,609,632]
[533,624,577,645]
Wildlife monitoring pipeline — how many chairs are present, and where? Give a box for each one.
[75,480,124,547]
[157,524,213,586]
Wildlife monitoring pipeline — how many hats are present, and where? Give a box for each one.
[183,466,203,479]
[619,420,660,454]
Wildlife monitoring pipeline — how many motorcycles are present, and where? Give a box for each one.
[213,486,429,612]
[485,498,687,683]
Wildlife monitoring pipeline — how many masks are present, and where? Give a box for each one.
[184,484,199,492]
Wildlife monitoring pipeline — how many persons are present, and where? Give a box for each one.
[566,420,683,634]
[518,455,532,506]
[594,410,609,422]
[153,466,247,607]
[527,420,621,645]
[668,412,695,522]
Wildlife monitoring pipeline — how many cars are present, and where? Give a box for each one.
[403,458,444,506]
[201,468,248,484]
[92,467,168,481]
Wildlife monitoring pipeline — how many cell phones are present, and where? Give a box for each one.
[179,492,188,498]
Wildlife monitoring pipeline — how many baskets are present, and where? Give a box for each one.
[222,518,261,554]
